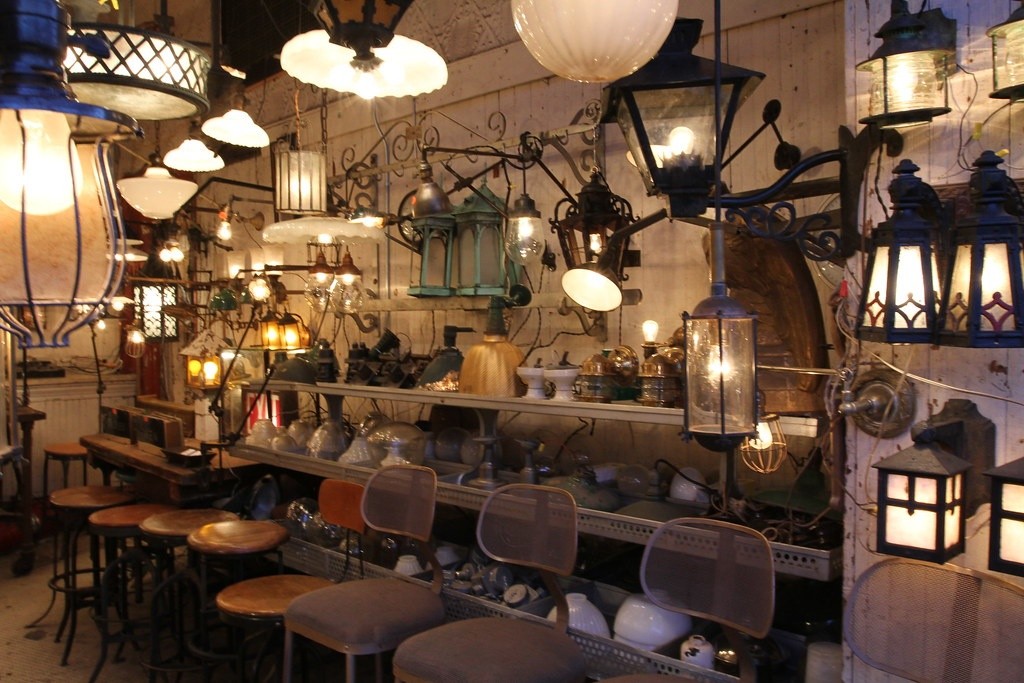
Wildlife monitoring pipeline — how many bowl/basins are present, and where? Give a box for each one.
[248,340,779,676]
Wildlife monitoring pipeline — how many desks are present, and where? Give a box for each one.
[78,432,260,510]
[5,402,46,549]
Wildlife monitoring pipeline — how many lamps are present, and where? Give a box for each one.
[593,16,905,268]
[854,0,957,130]
[511,0,679,83]
[280,0,449,101]
[201,82,272,147]
[271,86,330,215]
[66,0,214,119]
[164,116,226,172]
[560,208,667,315]
[269,128,381,224]
[984,0,1024,104]
[156,266,238,390]
[345,205,404,231]
[677,283,760,512]
[0,0,145,349]
[105,237,150,262]
[123,323,149,360]
[215,200,265,241]
[739,387,788,475]
[505,161,547,268]
[931,149,1024,349]
[853,158,955,346]
[412,145,521,219]
[159,236,184,263]
[980,455,1024,579]
[117,119,199,222]
[868,398,997,565]
[243,238,366,351]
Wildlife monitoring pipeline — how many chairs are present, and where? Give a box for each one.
[278,465,446,683]
[843,556,1024,683]
[599,517,777,683]
[390,482,587,683]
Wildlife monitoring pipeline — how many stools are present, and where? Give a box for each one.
[185,519,290,683]
[25,486,130,670]
[86,501,175,683]
[138,511,242,683]
[40,440,93,503]
[213,573,341,683]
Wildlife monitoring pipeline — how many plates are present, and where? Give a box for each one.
[160,445,216,467]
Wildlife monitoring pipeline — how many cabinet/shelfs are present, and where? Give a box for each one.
[8,372,139,501]
[226,380,845,683]
